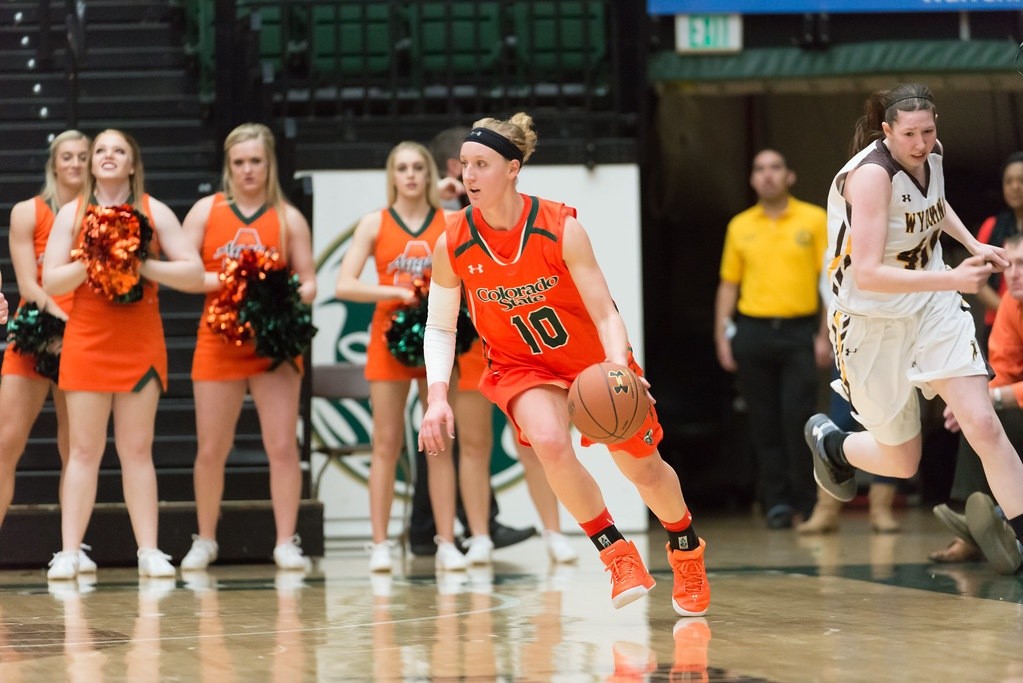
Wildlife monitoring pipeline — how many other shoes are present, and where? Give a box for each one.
[964,492,1021,575]
[765,504,792,530]
[930,538,972,564]
[934,503,975,546]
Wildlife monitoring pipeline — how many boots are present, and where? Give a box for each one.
[795,490,846,534]
[868,483,899,532]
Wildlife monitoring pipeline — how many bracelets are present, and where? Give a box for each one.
[993,386,1003,412]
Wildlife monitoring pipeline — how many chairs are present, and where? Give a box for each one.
[311,364,411,551]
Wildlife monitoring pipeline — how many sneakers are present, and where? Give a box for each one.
[181,535,218,569]
[463,535,495,563]
[666,537,711,616]
[804,412,857,502]
[272,535,306,568]
[540,531,577,562]
[138,548,176,576]
[604,640,658,683]
[432,536,469,571]
[600,540,656,608]
[364,541,392,570]
[48,551,77,578]
[79,543,97,573]
[671,618,711,683]
[538,566,573,593]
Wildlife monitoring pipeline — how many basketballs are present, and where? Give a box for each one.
[566,362,652,444]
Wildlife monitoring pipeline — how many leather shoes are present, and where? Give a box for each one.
[488,522,537,547]
[411,538,466,558]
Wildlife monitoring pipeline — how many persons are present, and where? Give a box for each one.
[42,126,204,579]
[716,146,833,526]
[0,130,96,572]
[439,174,579,562]
[805,85,1023,542]
[419,114,709,615]
[801,476,900,533]
[928,159,1023,573]
[335,144,470,573]
[410,129,536,558]
[182,124,317,570]
